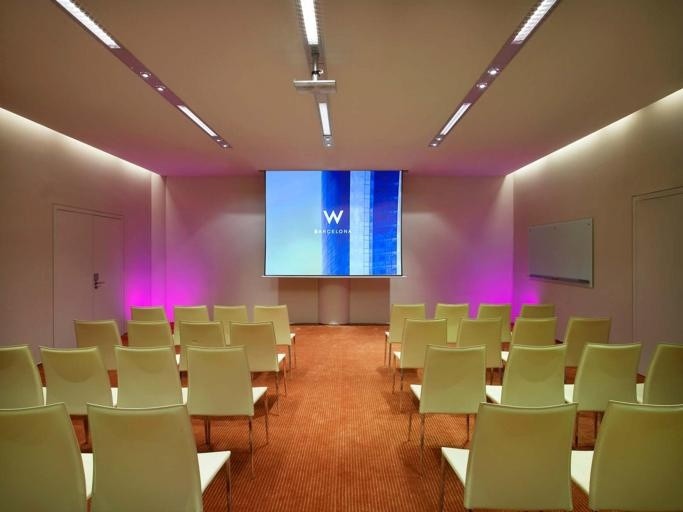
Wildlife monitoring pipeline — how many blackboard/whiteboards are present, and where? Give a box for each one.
[527,218,593,289]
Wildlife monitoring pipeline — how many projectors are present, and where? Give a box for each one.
[293,80,336,90]
